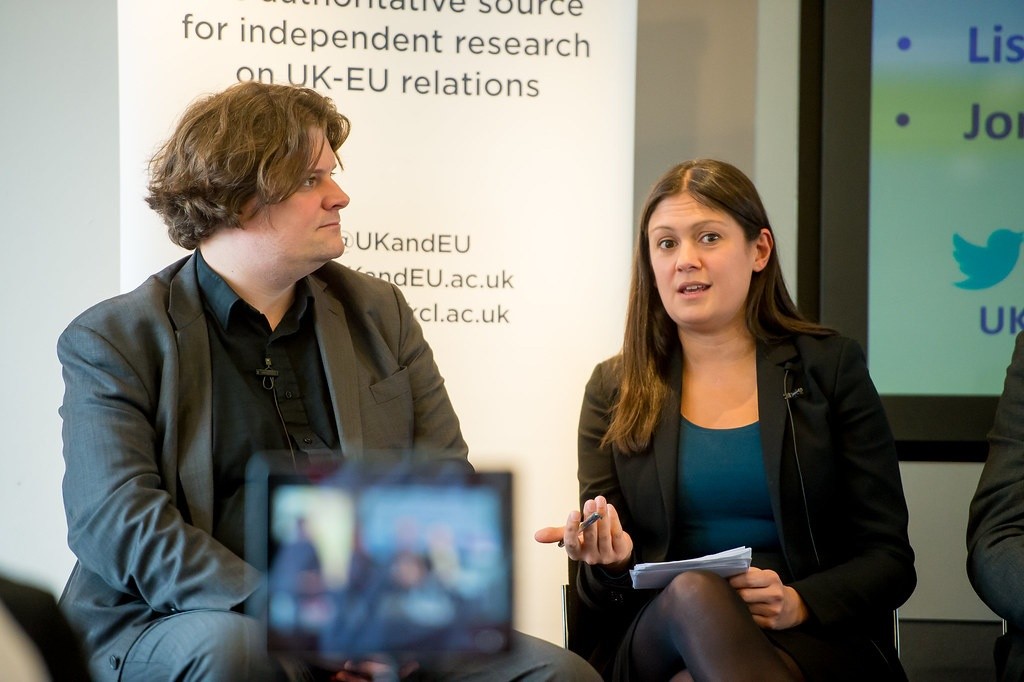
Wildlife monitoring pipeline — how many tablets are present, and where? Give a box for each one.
[244,454,514,658]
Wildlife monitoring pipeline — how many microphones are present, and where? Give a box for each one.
[784,362,794,371]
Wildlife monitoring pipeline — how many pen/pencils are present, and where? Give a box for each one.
[557,512,604,549]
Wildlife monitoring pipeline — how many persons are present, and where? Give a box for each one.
[274,513,474,630]
[57,82,604,682]
[535,160,917,682]
[966,330,1023,682]
